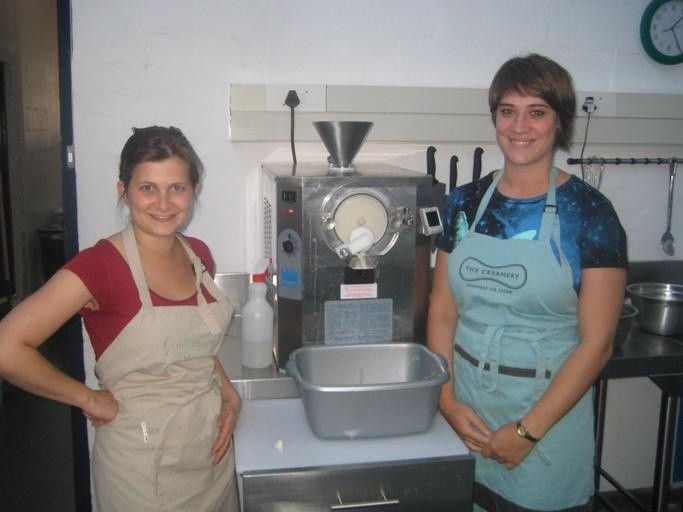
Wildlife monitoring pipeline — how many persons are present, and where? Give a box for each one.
[0,120,243,512]
[426,50,630,512]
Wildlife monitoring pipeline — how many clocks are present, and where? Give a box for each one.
[639,0,683,66]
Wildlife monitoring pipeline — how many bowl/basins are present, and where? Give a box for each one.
[615,282,683,350]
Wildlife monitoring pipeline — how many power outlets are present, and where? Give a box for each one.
[264,83,327,112]
[573,89,617,117]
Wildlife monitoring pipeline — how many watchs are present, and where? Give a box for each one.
[511,419,539,445]
